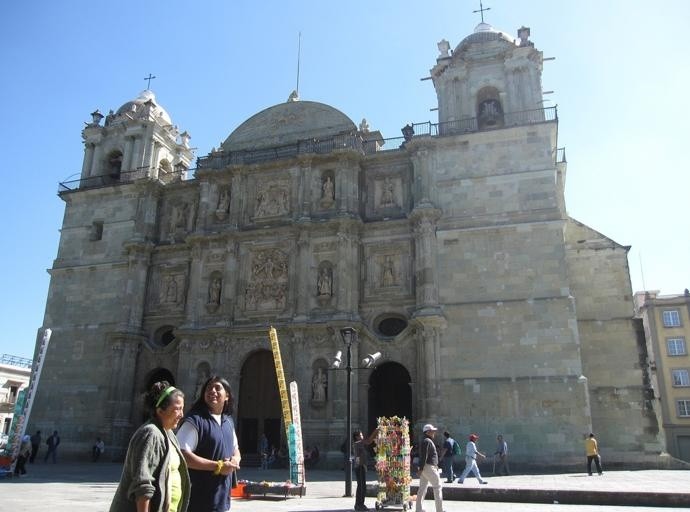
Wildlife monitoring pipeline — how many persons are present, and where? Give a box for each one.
[318,268,332,296]
[208,277,220,304]
[91,437,104,458]
[493,434,511,475]
[312,368,327,401]
[215,188,230,213]
[254,432,312,469]
[341,423,462,512]
[382,255,395,288]
[166,275,177,302]
[359,118,370,140]
[175,376,242,512]
[109,380,192,512]
[458,434,488,484]
[584,432,604,476]
[381,176,392,203]
[323,175,334,200]
[15,435,32,478]
[44,430,61,465]
[30,430,42,463]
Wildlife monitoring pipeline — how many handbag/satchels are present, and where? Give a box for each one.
[454,441,461,455]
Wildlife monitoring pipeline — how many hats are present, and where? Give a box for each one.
[423,424,437,432]
[470,435,479,439]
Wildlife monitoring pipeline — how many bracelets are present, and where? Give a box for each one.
[213,460,224,476]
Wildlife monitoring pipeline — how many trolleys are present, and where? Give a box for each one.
[372,471,423,512]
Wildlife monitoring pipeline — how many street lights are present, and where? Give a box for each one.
[330,325,383,500]
